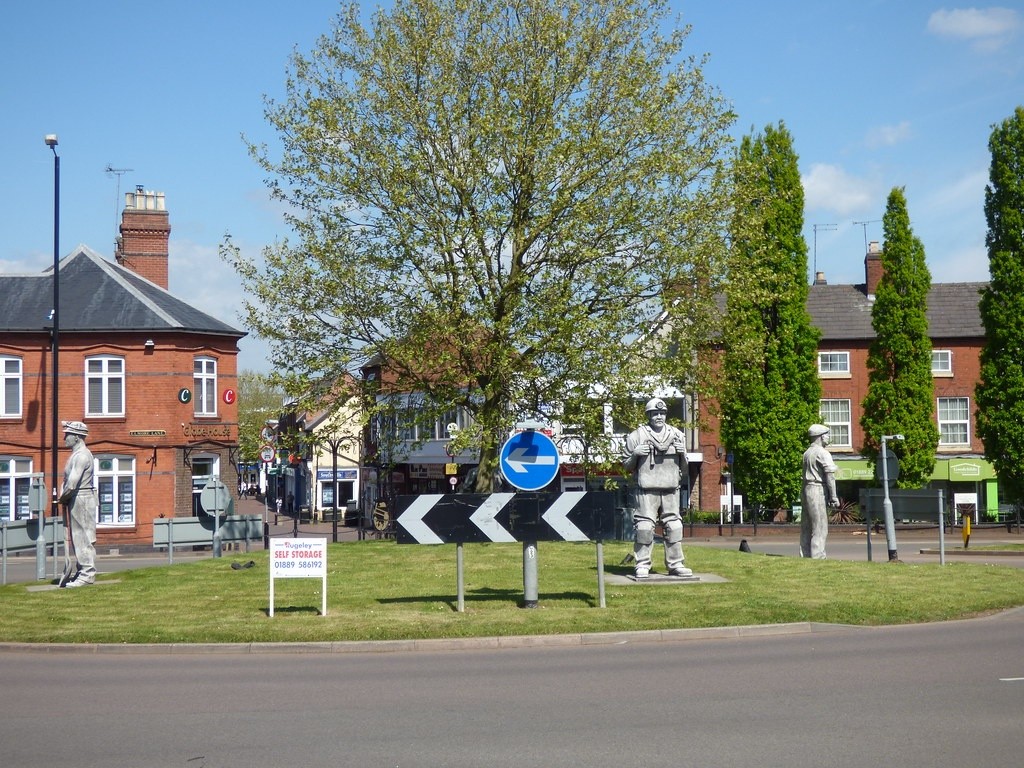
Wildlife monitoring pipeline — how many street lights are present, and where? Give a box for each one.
[44,132,61,555]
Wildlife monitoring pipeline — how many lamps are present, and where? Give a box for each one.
[145,339,154,348]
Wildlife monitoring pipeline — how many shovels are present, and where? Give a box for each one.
[58,502,72,587]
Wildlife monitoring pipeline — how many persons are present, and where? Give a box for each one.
[57,420,98,589]
[798,424,842,560]
[622,396,697,577]
[238,480,261,500]
[276,495,283,513]
[286,491,295,514]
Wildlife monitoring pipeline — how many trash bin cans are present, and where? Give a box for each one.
[299,504,311,521]
